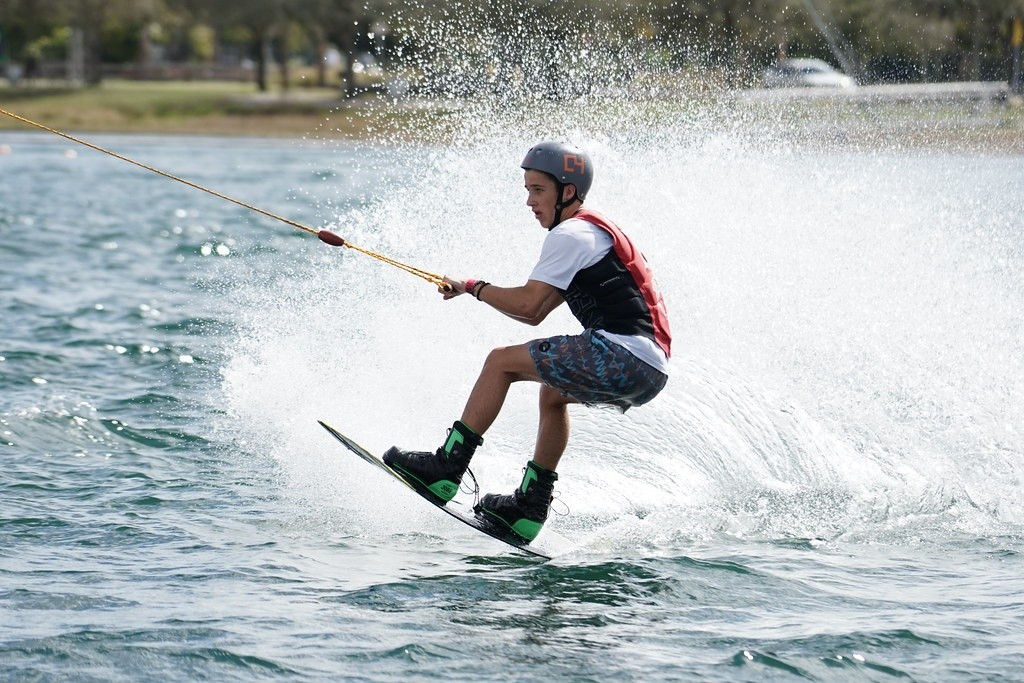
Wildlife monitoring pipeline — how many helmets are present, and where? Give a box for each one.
[520,140,593,200]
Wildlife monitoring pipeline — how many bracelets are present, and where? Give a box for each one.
[465,278,491,301]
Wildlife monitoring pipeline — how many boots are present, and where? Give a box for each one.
[382,419,483,508]
[472,461,558,545]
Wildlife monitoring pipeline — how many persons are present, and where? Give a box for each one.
[382,140,672,545]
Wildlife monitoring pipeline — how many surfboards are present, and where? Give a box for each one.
[316,419,555,560]
